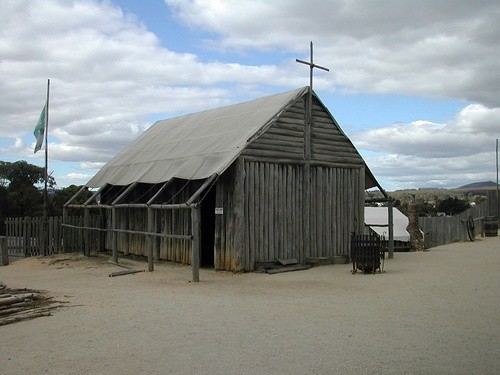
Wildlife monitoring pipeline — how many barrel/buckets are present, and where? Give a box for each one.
[484,216,498,237]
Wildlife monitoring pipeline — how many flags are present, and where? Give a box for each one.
[33,106,46,153]
